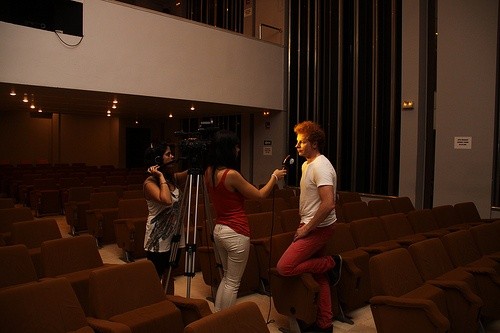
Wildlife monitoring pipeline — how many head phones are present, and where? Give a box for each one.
[152,149,162,164]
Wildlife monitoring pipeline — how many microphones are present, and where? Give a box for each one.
[282,155,291,170]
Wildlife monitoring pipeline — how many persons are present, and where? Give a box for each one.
[203,131,287,316]
[143,137,198,279]
[275,121,343,333]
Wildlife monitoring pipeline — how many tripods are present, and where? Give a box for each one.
[161,166,223,297]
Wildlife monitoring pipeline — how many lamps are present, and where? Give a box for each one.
[402,101,414,110]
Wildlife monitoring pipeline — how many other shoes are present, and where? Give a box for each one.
[302,322,334,333]
[329,254,342,286]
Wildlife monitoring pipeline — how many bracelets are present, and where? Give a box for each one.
[271,173,278,184]
[160,181,167,185]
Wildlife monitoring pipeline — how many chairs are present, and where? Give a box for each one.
[0,163,500,333]
